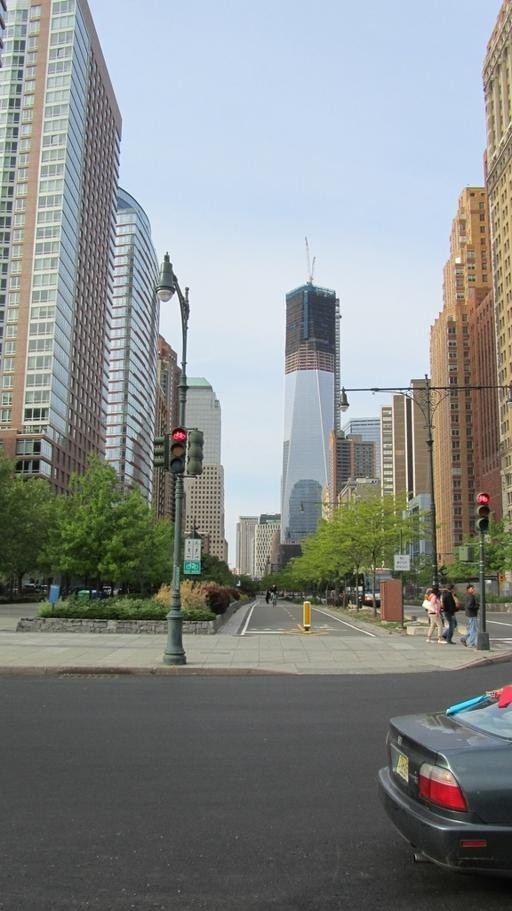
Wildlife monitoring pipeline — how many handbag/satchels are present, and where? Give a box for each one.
[422,600,433,613]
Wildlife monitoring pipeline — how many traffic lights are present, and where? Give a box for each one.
[152,428,186,475]
[475,493,491,531]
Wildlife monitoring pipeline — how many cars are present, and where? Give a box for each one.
[376,684,512,881]
[23,582,48,592]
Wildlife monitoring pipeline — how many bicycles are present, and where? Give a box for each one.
[271,593,278,609]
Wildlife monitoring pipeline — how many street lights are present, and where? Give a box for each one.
[338,385,512,626]
[154,250,191,666]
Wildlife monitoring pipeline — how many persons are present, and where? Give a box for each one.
[423,582,482,649]
[265,584,279,608]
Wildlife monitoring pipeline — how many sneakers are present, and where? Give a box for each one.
[460,639,467,647]
[425,635,454,644]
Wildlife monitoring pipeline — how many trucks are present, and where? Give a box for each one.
[346,567,393,606]
